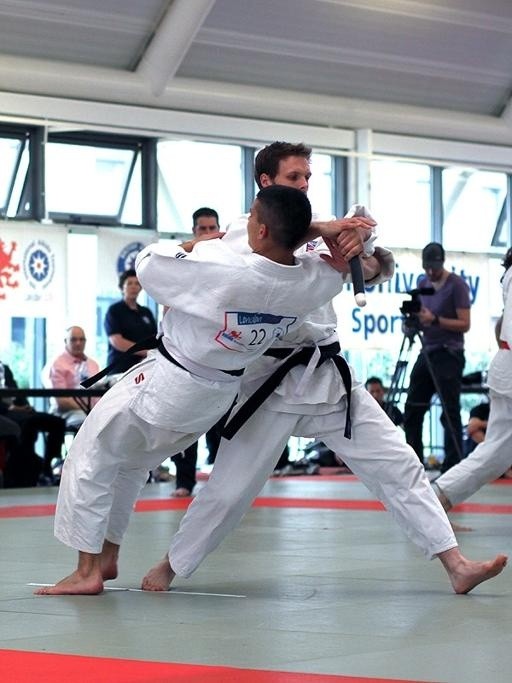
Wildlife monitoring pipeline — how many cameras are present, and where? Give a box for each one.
[402,288,435,314]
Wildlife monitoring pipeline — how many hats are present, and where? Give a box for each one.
[422,241,445,271]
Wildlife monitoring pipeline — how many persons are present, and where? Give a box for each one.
[172,208,232,496]
[140,138,507,595]
[398,242,468,467]
[0,271,509,489]
[32,191,375,599]
[427,248,507,508]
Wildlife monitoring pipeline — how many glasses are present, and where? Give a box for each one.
[68,336,85,342]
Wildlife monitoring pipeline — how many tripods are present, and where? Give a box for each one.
[385,339,462,462]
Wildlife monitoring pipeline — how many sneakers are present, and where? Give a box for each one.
[300,457,320,475]
[274,463,305,476]
